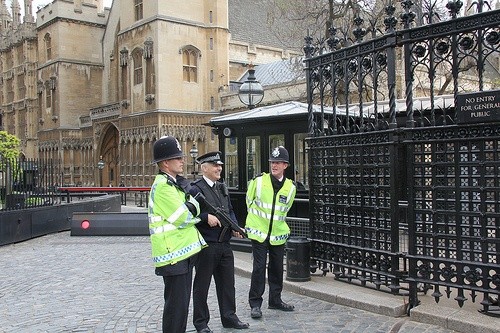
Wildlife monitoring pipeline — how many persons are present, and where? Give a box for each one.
[245,146,296,318]
[147,136,210,333]
[189,151,250,333]
[62,180,126,205]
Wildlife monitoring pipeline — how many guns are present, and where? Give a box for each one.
[194,191,246,242]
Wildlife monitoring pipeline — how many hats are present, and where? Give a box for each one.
[196,151,224,165]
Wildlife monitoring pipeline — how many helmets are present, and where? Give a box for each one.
[268,145,289,163]
[153,135,183,163]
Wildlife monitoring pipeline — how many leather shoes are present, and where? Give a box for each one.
[268,300,296,311]
[196,326,213,333]
[251,307,262,318]
[223,320,250,329]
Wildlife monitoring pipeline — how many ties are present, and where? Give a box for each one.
[213,183,219,198]
